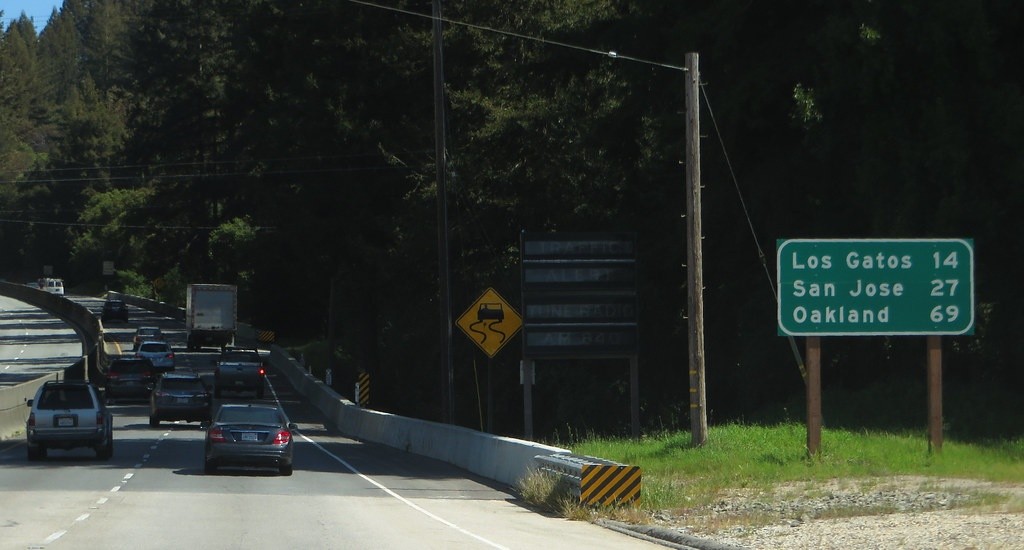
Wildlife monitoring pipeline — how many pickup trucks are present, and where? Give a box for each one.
[215,347,265,397]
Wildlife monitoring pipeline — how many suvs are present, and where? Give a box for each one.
[136,341,176,372]
[148,374,213,426]
[26,379,113,462]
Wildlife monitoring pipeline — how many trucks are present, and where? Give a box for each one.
[182,284,238,352]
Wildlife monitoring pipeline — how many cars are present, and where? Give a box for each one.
[103,356,159,402]
[101,301,128,323]
[200,404,296,476]
[133,326,165,349]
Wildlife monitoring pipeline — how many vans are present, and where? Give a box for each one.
[39,279,64,296]
